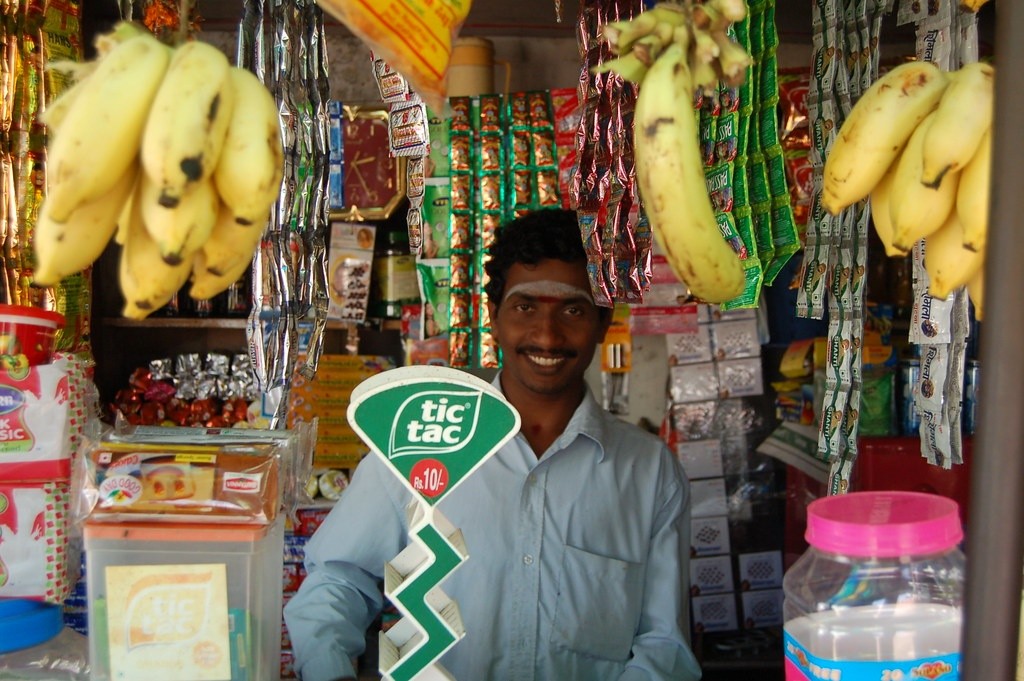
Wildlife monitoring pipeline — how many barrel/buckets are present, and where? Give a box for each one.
[0,304,67,369]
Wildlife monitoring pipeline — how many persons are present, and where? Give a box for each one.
[283,208,702,681]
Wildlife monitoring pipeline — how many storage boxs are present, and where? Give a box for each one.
[772,329,898,424]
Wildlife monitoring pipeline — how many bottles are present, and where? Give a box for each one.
[782,490,965,680]
[369,231,421,319]
[163,272,247,317]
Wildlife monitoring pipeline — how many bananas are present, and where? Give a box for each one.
[631,41,745,304]
[23,22,284,320]
[820,59,992,320]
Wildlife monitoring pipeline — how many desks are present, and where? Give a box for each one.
[753,421,974,555]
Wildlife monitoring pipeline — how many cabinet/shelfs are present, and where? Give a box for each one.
[93,316,401,427]
